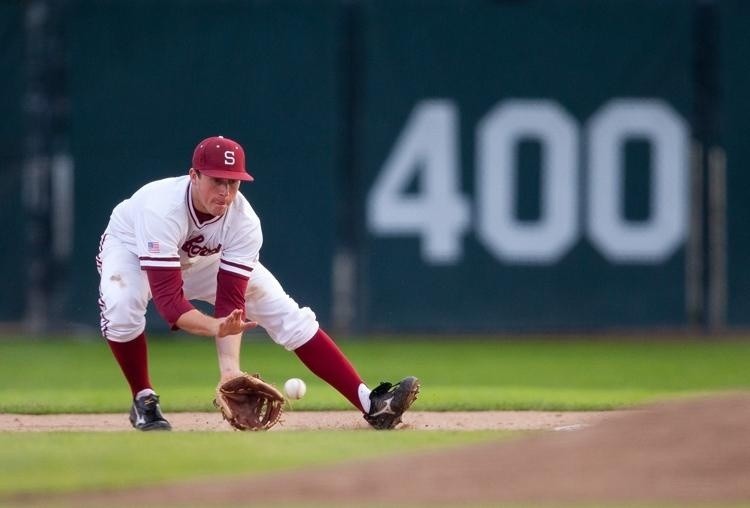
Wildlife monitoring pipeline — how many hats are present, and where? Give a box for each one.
[192,131,255,183]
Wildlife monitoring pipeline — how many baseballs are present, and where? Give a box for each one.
[285,378,307,399]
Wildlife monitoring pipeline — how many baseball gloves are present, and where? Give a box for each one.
[216,374,285,432]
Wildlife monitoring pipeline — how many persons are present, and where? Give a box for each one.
[96,136,420,430]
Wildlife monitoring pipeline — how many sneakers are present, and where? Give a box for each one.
[126,392,172,432]
[362,374,421,432]
